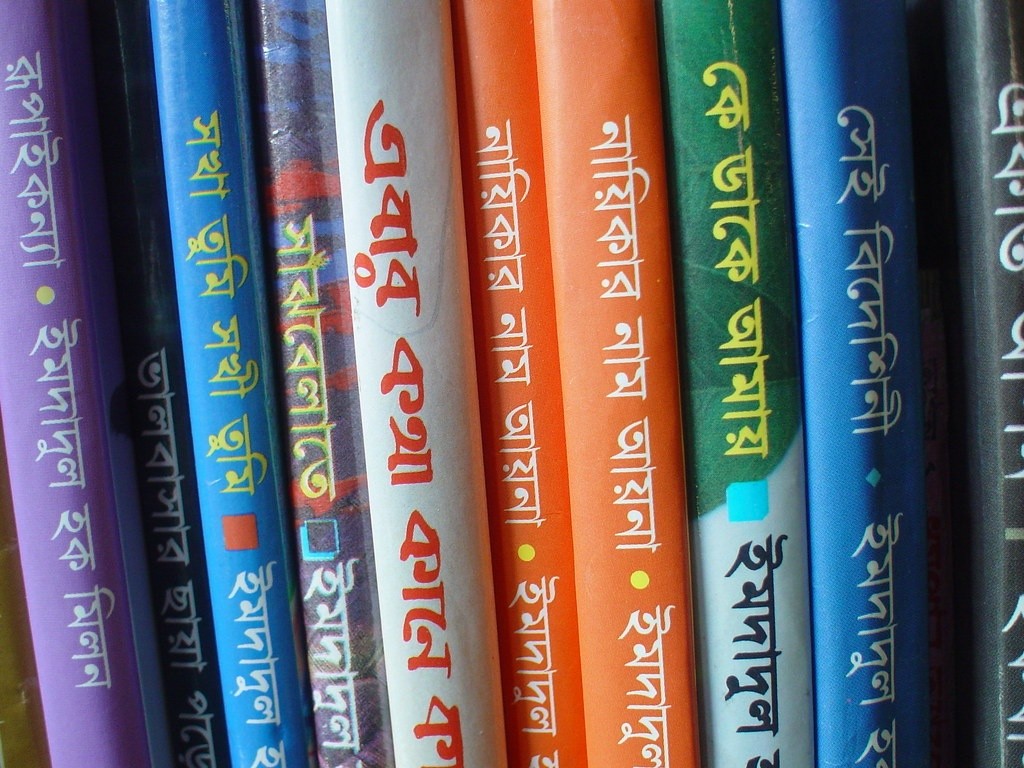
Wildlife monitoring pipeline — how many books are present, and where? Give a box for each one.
[0,0,1024,768]
